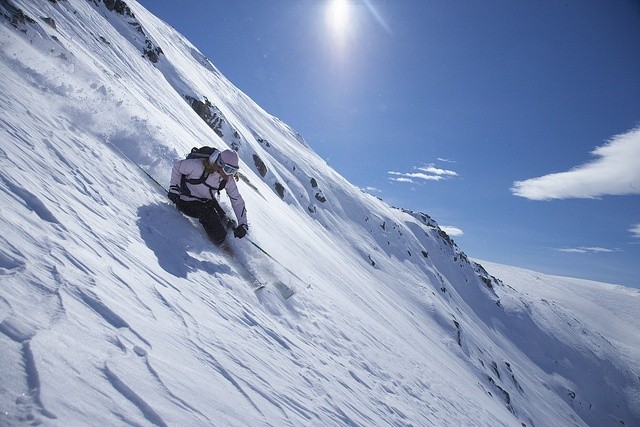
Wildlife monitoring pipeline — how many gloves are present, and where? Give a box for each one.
[168,192,180,203]
[234,224,248,239]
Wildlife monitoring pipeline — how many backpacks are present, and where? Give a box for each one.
[180,146,227,202]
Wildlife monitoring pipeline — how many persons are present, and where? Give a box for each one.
[168,147,249,247]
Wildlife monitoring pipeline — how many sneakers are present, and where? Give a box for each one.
[218,242,230,253]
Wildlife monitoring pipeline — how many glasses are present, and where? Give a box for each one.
[218,157,239,176]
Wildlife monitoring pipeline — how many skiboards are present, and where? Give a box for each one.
[225,229,294,300]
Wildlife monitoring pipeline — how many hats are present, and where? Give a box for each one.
[217,149,238,167]
